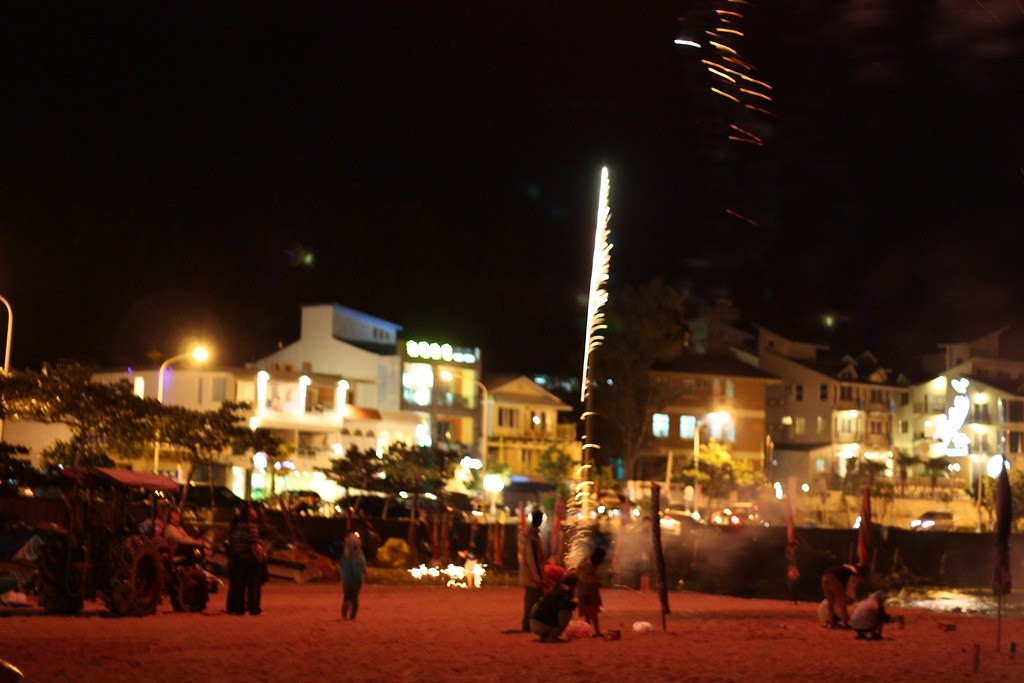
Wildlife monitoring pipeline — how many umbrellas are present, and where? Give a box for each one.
[785,496,800,607]
[993,462,1012,651]
[858,485,872,570]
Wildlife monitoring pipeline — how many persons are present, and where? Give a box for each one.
[450,542,479,565]
[226,509,269,615]
[340,534,367,621]
[818,563,896,639]
[166,512,207,570]
[520,511,606,642]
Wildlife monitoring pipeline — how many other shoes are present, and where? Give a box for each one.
[540,632,561,641]
[857,633,866,639]
[872,633,883,640]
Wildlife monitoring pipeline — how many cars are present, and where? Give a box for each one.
[176,482,266,539]
[913,510,955,535]
